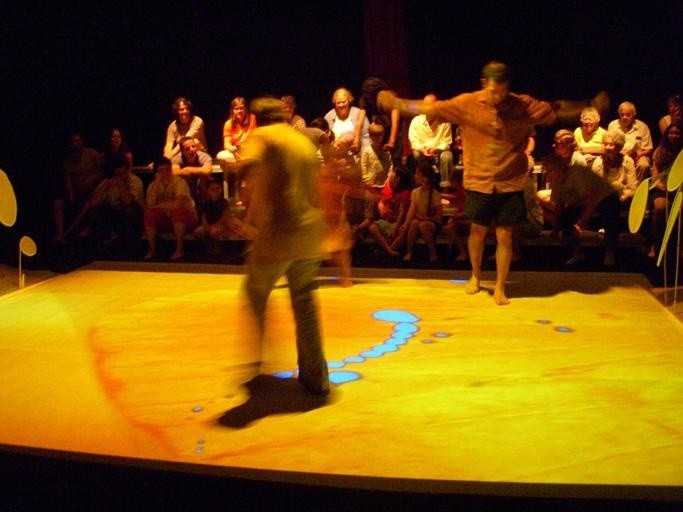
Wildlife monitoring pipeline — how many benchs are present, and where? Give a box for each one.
[36,122,681,274]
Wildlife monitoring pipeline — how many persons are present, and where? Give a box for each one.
[215,97,347,395]
[52,62,683,306]
